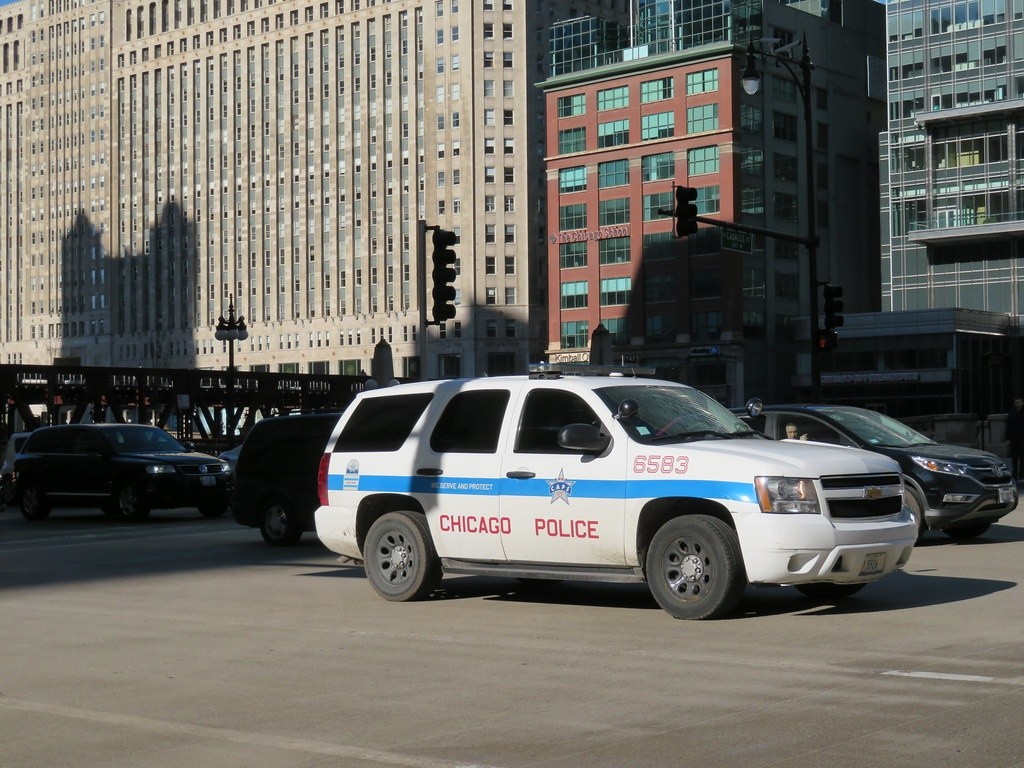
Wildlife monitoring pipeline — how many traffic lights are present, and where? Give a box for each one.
[675,185,698,237]
[819,332,839,349]
[824,285,844,330]
[431,229,457,324]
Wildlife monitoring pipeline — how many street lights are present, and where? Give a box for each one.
[214,293,250,448]
[742,29,827,403]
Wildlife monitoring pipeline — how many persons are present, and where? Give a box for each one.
[1001,396,1024,483]
[784,420,814,441]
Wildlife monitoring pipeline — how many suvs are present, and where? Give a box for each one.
[1,429,32,505]
[230,411,353,547]
[727,404,1019,544]
[312,362,918,621]
[12,421,234,523]
